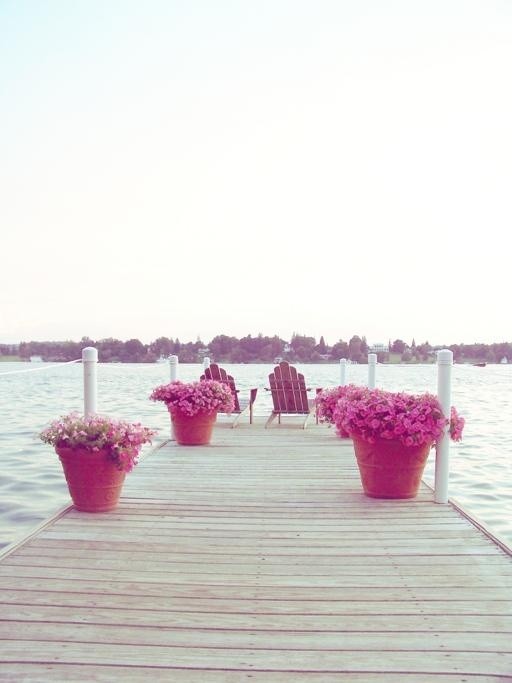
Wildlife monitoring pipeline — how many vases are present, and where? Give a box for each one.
[54,445,130,513]
[352,436,431,500]
[170,413,217,446]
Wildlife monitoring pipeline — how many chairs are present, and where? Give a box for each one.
[263,360,323,430]
[199,363,258,429]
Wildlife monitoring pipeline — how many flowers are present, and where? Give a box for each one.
[36,410,158,472]
[148,378,237,418]
[314,380,464,447]
